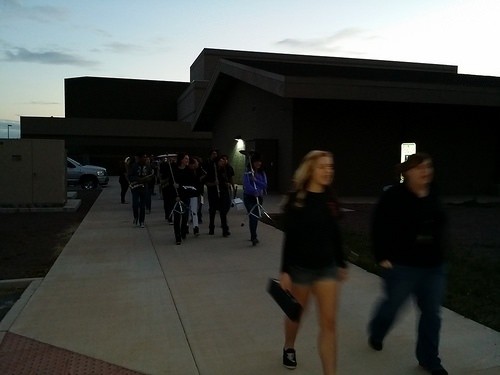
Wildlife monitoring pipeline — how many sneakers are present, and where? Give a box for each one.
[283,347,297,369]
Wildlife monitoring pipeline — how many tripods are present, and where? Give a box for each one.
[241,154,274,226]
[166,153,196,219]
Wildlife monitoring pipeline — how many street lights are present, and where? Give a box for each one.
[7,125,12,138]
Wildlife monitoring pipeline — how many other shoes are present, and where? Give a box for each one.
[209,225,214,234]
[141,222,144,228]
[170,222,174,225]
[133,218,138,224]
[223,226,229,236]
[194,227,199,235]
[185,226,189,233]
[176,238,180,244]
[182,231,185,238]
[252,236,259,246]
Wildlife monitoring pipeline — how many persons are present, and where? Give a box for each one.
[242,155,268,245]
[279,150,351,375]
[119,150,236,245]
[362,153,448,375]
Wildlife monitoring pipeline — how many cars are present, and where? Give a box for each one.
[65,155,110,195]
